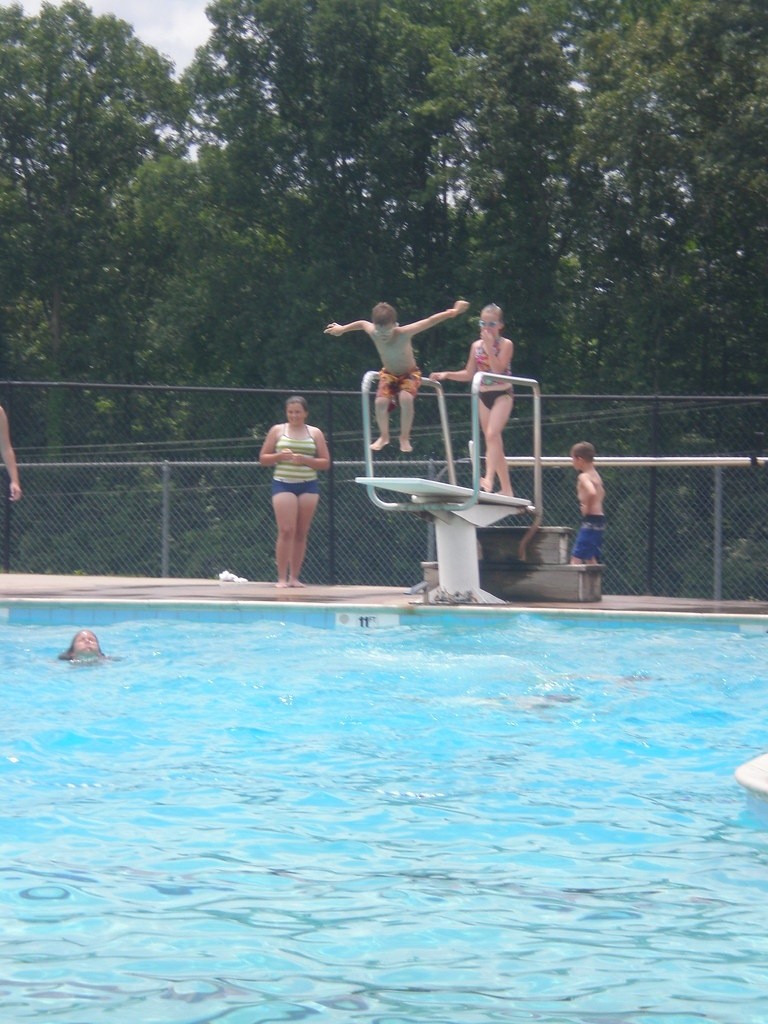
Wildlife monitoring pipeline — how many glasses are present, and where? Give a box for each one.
[373,325,394,336]
[478,320,501,328]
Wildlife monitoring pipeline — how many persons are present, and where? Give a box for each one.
[72,630,100,653]
[570,442,606,563]
[0,407,22,500]
[260,397,331,589]
[323,300,471,452]
[429,304,514,497]
